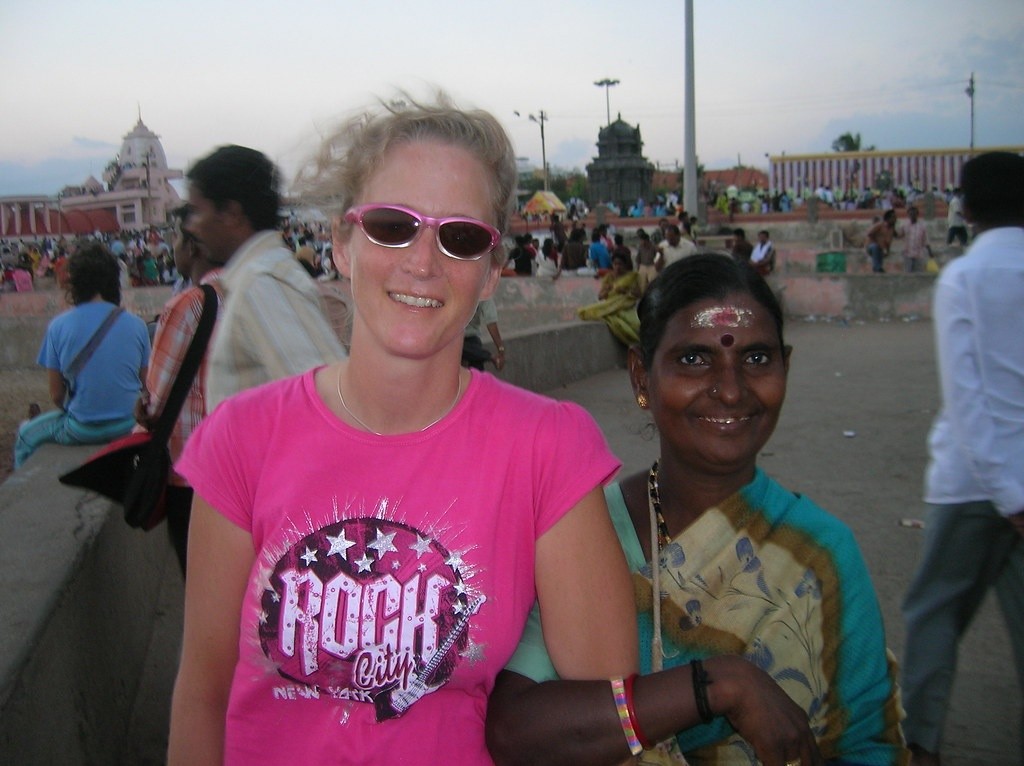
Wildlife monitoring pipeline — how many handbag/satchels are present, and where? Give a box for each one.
[57,284,220,530]
[925,255,939,271]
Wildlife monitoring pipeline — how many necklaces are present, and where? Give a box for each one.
[654,470,661,550]
[648,460,671,547]
[336,362,461,435]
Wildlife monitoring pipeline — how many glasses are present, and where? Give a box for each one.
[344,203,506,263]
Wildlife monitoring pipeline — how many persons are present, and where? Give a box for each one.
[485,255,918,766]
[904,150,1024,766]
[0,146,972,586]
[168,103,641,766]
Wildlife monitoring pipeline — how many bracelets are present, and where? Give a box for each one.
[498,346,504,351]
[690,660,715,724]
[608,670,656,756]
